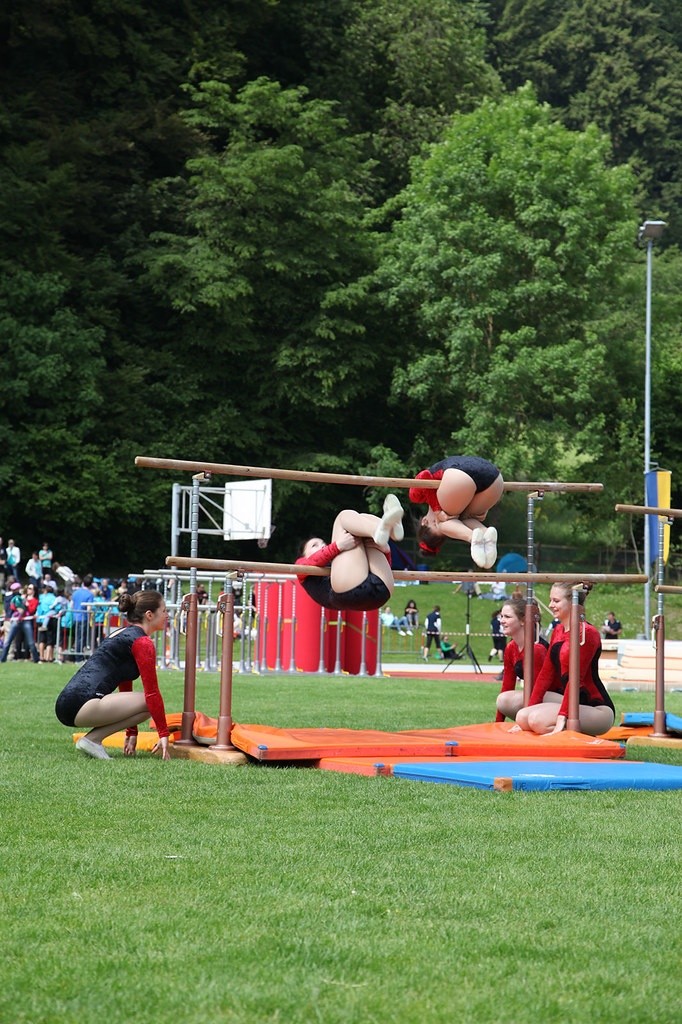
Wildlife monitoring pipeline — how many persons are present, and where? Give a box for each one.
[0,537,144,664]
[55,589,171,760]
[381,606,413,636]
[407,456,505,568]
[601,612,622,639]
[195,584,208,605]
[516,582,616,738]
[422,605,444,662]
[296,493,404,611]
[488,610,506,661]
[452,569,482,599]
[436,636,465,660]
[546,615,561,637]
[218,585,256,617]
[495,591,551,733]
[483,582,507,601]
[405,600,419,629]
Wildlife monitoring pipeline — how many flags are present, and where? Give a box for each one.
[646,472,671,567]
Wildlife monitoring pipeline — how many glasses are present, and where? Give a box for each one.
[27,587,32,590]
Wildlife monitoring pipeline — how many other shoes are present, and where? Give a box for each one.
[76,737,113,760]
[383,494,404,541]
[407,631,413,636]
[374,508,403,546]
[483,527,497,569]
[500,660,503,664]
[38,626,48,632]
[399,631,406,636]
[488,656,492,662]
[471,528,486,567]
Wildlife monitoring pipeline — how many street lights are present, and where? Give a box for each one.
[646,220,677,740]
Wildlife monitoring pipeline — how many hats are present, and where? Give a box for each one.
[10,583,20,590]
